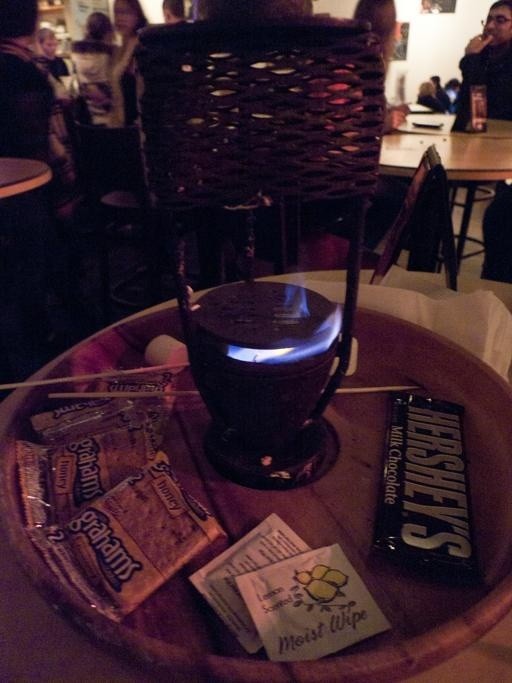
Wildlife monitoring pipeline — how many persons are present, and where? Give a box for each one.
[38,0,208,225]
[2,0,38,225]
[417,70,459,114]
[449,0,511,279]
[346,0,405,131]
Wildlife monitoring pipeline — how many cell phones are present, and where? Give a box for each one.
[412,121,444,129]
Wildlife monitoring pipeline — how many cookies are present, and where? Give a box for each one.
[12,414,161,535]
[50,371,180,430]
[30,396,145,445]
[20,449,228,622]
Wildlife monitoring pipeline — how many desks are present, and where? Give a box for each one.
[4,267,512,682]
[318,105,512,291]
[4,154,52,200]
